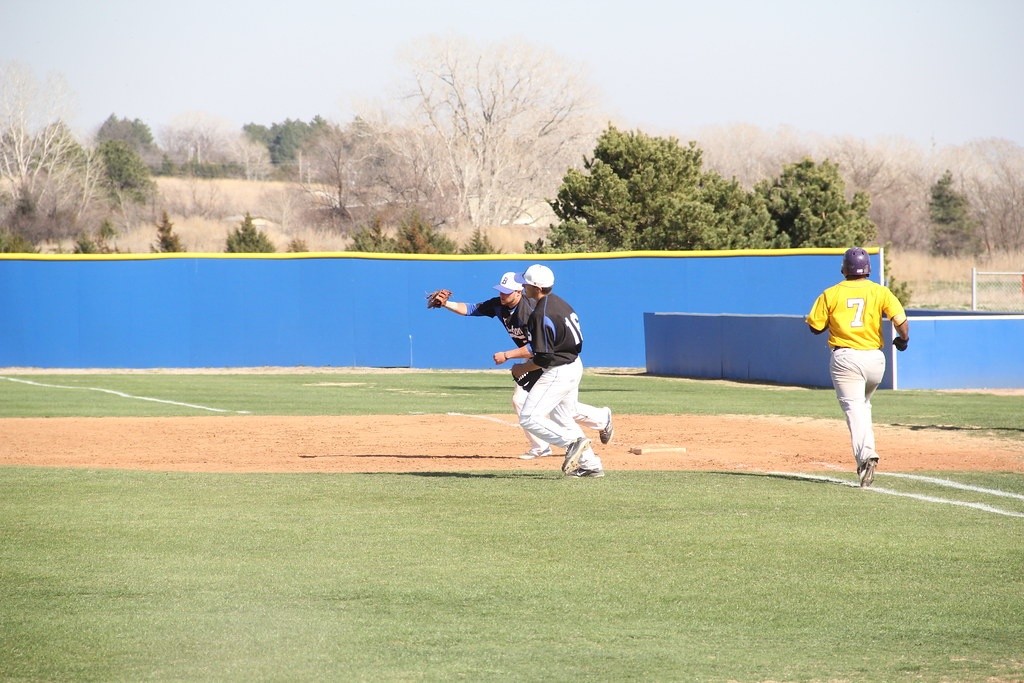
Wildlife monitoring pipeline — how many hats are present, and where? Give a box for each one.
[514,264,554,289]
[491,271,524,296]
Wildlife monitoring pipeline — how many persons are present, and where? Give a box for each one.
[512,264,605,477]
[805,248,910,486]
[427,272,612,459]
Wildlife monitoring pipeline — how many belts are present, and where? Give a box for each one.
[833,345,850,352]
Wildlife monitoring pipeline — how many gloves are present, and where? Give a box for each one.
[892,337,909,352]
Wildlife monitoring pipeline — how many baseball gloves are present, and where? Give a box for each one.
[509,360,544,393]
[424,289,452,309]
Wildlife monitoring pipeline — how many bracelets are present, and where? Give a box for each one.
[504,352,508,361]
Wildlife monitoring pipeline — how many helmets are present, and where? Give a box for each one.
[839,245,872,278]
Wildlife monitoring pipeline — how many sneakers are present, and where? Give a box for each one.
[517,446,553,459]
[857,456,878,487]
[564,467,605,479]
[600,407,613,444]
[562,437,592,473]
